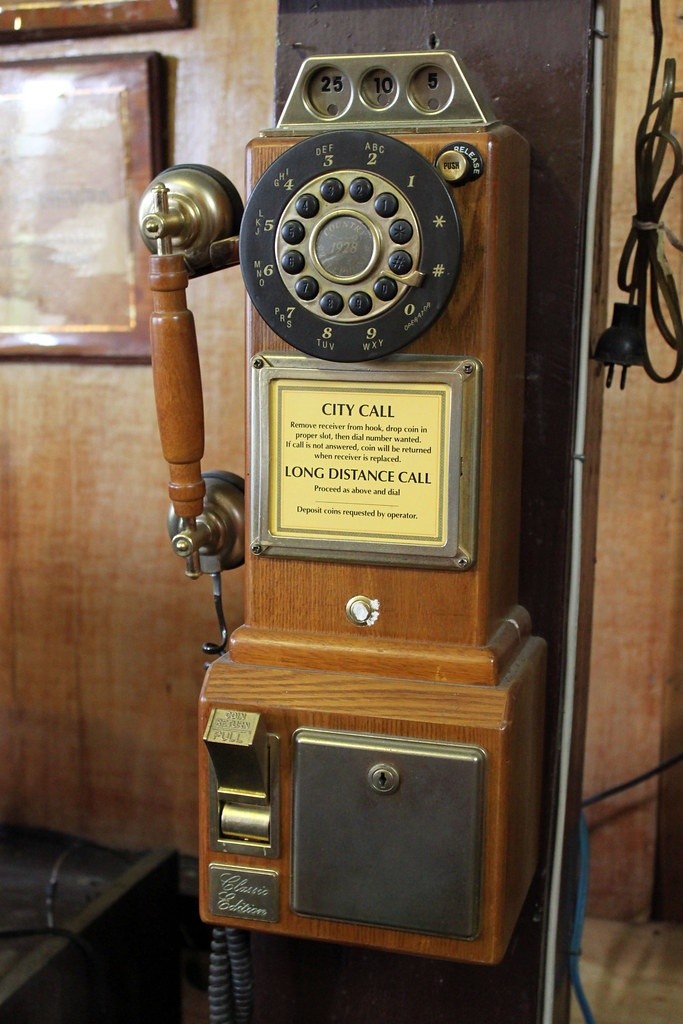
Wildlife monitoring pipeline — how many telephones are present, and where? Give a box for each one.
[136,52,553,966]
